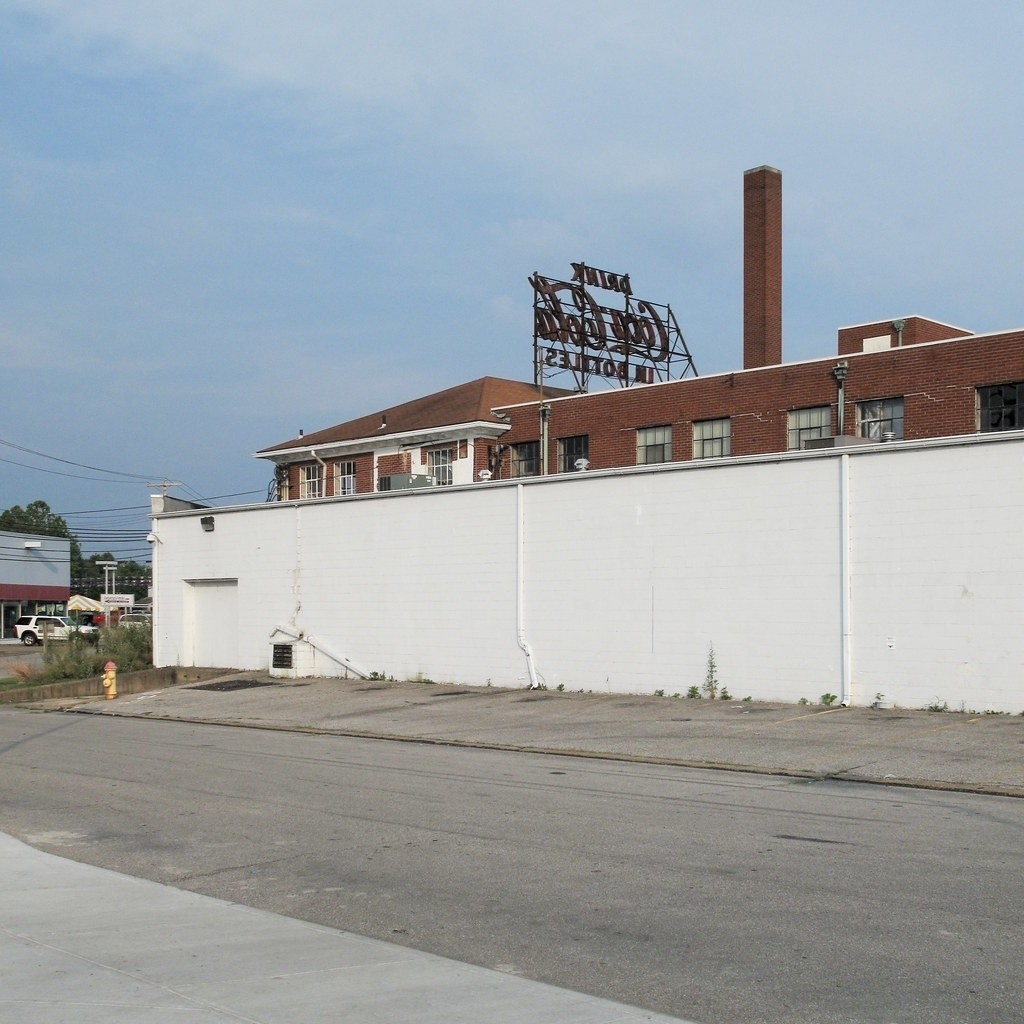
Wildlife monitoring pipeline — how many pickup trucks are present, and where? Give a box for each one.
[92,609,125,627]
[14,614,100,646]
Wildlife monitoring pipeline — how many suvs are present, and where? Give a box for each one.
[118,614,152,631]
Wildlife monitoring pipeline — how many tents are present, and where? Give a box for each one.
[38,594,119,629]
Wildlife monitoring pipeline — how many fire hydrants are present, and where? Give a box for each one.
[100,661,118,700]
